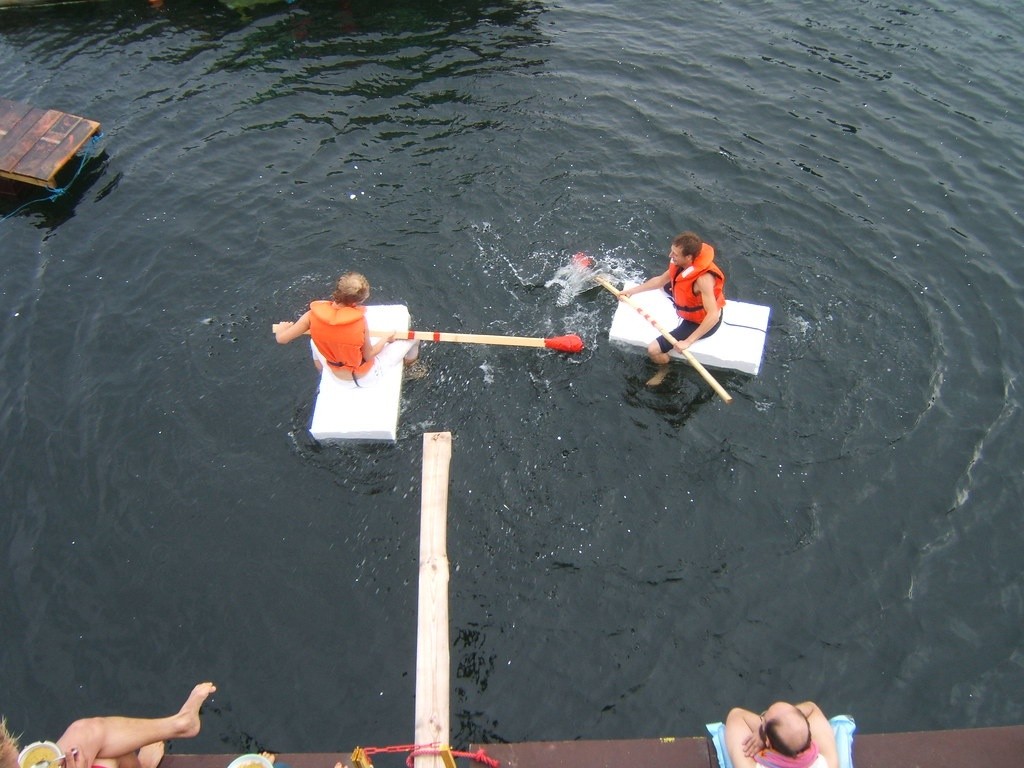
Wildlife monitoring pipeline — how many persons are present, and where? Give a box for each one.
[275,273,421,392]
[0,681,217,768]
[616,232,726,385]
[724,700,841,768]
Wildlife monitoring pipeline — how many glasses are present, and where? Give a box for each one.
[759,711,767,722]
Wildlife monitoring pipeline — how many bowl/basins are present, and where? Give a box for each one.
[17,740,62,768]
[228,754,274,768]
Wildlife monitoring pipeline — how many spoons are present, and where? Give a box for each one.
[31,749,77,768]
